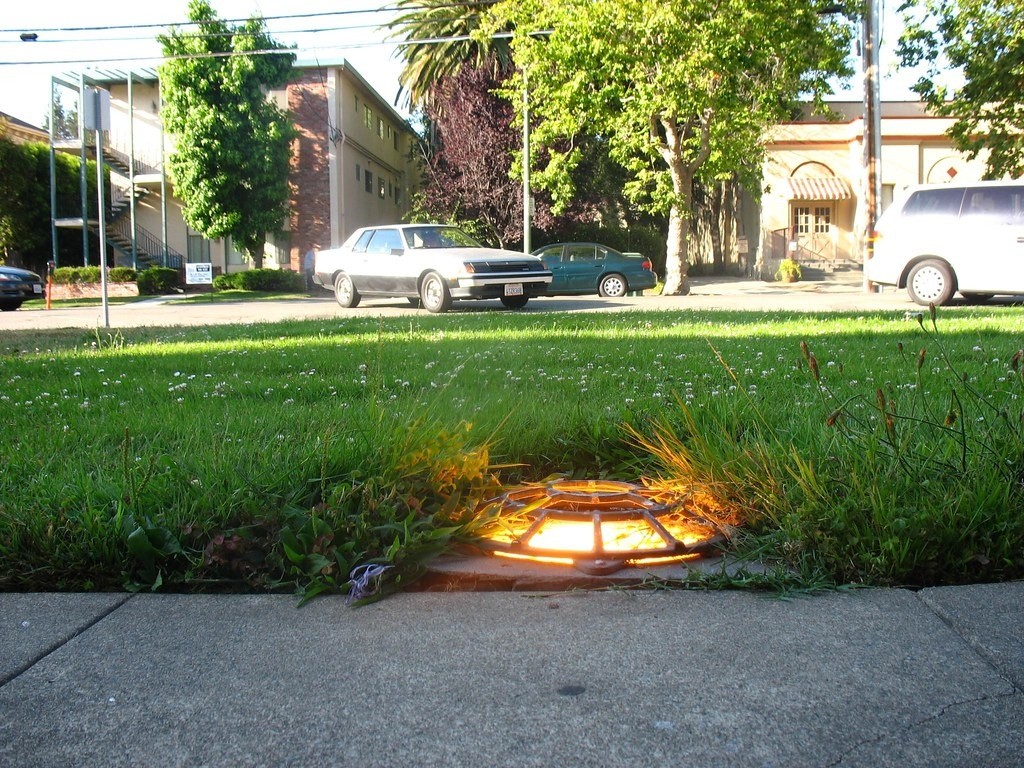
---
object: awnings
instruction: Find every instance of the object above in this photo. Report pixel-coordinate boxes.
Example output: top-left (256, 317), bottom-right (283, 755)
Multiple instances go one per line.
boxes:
top-left (786, 177), bottom-right (851, 200)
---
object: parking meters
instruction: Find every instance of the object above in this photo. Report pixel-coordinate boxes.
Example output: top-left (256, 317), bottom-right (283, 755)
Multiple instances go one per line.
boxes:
top-left (47, 260), bottom-right (56, 309)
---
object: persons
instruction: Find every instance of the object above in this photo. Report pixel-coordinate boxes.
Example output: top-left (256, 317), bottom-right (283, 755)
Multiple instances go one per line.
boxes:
top-left (303, 242), bottom-right (315, 292)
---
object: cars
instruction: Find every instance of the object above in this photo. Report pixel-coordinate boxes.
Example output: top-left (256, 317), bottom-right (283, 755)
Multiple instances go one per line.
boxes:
top-left (0, 265), bottom-right (46, 311)
top-left (868, 180), bottom-right (1024, 307)
top-left (529, 243), bottom-right (657, 298)
top-left (312, 224), bottom-right (553, 313)
